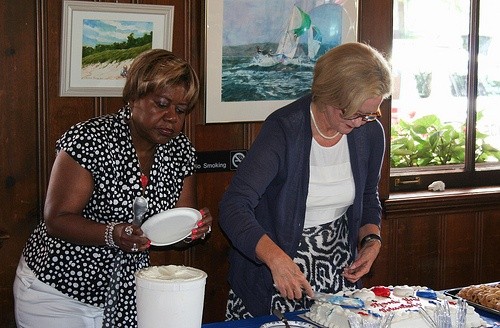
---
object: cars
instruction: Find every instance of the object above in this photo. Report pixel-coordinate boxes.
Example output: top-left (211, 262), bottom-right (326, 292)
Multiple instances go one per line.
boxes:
top-left (388, 36), bottom-right (500, 161)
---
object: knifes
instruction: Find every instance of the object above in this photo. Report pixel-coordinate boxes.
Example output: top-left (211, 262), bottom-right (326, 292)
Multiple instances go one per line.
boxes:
top-left (273, 284), bottom-right (362, 307)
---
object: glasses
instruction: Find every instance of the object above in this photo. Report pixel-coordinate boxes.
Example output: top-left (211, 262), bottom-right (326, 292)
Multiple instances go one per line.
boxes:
top-left (338, 103), bottom-right (381, 122)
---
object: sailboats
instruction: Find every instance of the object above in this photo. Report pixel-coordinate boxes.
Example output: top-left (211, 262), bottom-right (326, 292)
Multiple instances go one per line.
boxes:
top-left (255, 3), bottom-right (327, 72)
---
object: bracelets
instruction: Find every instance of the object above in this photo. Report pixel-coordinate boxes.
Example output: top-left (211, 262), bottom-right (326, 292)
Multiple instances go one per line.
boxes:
top-left (183, 236), bottom-right (192, 244)
top-left (104, 221), bottom-right (122, 249)
top-left (360, 234), bottom-right (382, 248)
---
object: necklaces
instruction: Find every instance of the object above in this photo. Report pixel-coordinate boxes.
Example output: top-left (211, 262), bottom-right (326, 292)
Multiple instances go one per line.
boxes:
top-left (310, 102), bottom-right (340, 139)
top-left (130, 130), bottom-right (158, 189)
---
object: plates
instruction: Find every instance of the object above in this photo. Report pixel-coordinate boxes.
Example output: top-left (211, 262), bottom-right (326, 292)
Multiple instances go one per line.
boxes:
top-left (140, 207), bottom-right (203, 246)
top-left (260, 320), bottom-right (318, 328)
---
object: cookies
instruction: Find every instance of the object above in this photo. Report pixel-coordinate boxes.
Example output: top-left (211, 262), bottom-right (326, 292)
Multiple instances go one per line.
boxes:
top-left (456, 283), bottom-right (500, 313)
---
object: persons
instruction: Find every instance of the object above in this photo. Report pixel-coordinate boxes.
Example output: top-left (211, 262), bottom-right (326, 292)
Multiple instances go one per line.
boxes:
top-left (13, 49), bottom-right (214, 328)
top-left (218, 42), bottom-right (392, 322)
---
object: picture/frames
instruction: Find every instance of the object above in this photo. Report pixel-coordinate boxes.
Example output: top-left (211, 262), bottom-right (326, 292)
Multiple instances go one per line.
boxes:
top-left (196, 0), bottom-right (363, 126)
top-left (58, 0), bottom-right (175, 97)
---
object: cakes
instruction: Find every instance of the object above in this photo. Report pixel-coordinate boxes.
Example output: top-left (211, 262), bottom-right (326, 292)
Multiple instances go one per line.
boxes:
top-left (305, 284), bottom-right (487, 328)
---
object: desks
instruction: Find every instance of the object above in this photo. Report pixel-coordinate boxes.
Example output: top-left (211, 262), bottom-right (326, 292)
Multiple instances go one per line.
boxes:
top-left (201, 283), bottom-right (500, 328)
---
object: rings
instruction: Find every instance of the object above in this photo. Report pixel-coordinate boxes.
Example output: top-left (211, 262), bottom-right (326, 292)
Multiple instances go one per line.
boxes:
top-left (130, 243), bottom-right (138, 253)
top-left (206, 224), bottom-right (212, 234)
top-left (201, 232), bottom-right (206, 240)
top-left (124, 225), bottom-right (134, 236)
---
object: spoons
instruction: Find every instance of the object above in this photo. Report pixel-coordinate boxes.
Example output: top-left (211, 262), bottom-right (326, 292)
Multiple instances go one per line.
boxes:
top-left (132, 197), bottom-right (148, 227)
